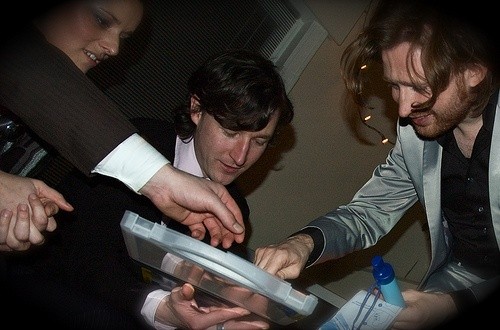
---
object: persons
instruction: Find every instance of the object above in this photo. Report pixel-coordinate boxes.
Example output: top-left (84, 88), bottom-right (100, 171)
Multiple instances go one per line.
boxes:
top-left (0, 0), bottom-right (248, 251)
top-left (18, 49), bottom-right (318, 330)
top-left (0, 0), bottom-right (145, 256)
top-left (252, 1), bottom-right (500, 329)
